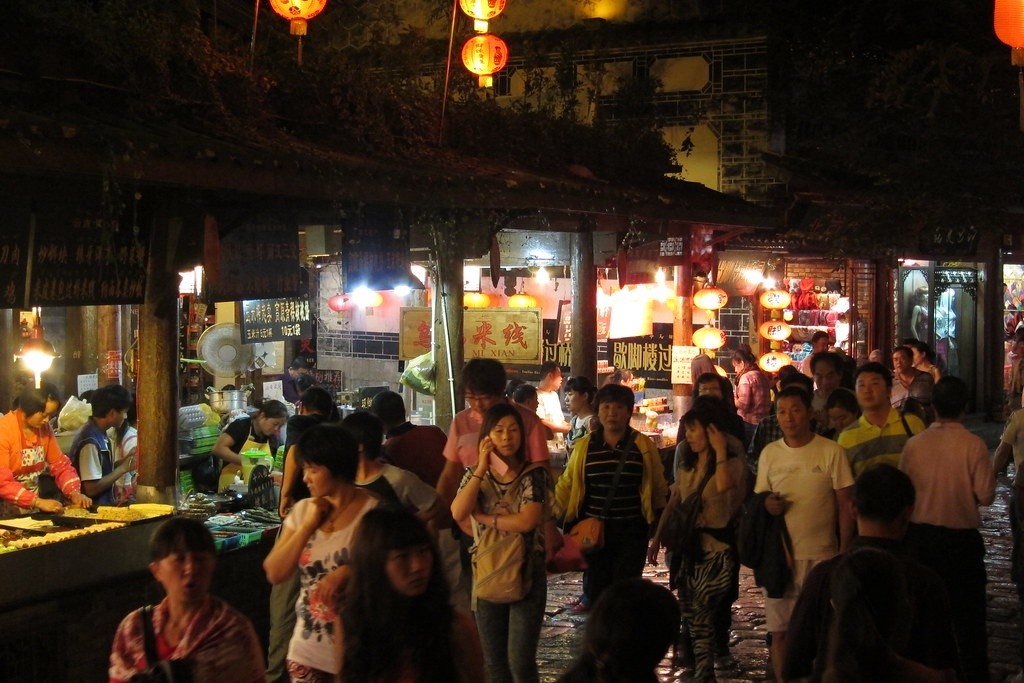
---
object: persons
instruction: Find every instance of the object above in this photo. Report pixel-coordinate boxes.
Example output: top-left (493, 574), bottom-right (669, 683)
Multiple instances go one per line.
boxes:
top-left (911, 286), bottom-right (959, 378)
top-left (264, 354), bottom-right (569, 683)
top-left (1004, 282), bottom-right (1024, 332)
top-left (109, 518), bottom-right (265, 683)
top-left (691, 332), bottom-right (1023, 683)
top-left (835, 313), bottom-right (865, 360)
top-left (556, 372), bottom-right (750, 682)
top-left (0, 382), bottom-right (286, 525)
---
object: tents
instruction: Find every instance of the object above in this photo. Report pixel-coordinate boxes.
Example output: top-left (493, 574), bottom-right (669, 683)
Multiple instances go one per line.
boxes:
top-left (464, 292), bottom-right (491, 309)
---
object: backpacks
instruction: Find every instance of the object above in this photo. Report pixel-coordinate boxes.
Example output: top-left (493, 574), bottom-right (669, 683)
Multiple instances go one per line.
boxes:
top-left (471, 464), bottom-right (550, 604)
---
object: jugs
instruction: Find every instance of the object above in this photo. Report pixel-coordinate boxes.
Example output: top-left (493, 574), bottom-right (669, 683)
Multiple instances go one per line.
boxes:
top-left (240, 447), bottom-right (274, 488)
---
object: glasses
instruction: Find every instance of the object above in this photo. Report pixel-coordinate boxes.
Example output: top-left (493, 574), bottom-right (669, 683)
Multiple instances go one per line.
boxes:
top-left (464, 394), bottom-right (495, 402)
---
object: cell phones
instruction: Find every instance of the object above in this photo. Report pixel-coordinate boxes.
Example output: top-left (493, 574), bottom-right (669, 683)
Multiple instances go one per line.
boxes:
top-left (484, 434), bottom-right (509, 476)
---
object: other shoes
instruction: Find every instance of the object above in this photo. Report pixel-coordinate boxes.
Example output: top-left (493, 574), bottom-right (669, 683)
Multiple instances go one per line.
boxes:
top-left (571, 602), bottom-right (589, 612)
top-left (565, 599), bottom-right (581, 607)
top-left (713, 654), bottom-right (735, 668)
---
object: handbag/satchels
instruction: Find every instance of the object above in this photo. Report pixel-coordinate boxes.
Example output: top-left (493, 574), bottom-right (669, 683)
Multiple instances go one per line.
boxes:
top-left (545, 518), bottom-right (604, 573)
top-left (659, 491), bottom-right (703, 549)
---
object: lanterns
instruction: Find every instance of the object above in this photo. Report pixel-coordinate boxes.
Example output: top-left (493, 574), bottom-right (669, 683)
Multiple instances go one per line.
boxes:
top-left (693, 285), bottom-right (727, 356)
top-left (508, 292), bottom-right (536, 309)
top-left (329, 292), bottom-right (383, 315)
top-left (270, 1), bottom-right (329, 64)
top-left (459, 0), bottom-right (509, 88)
top-left (759, 289), bottom-right (791, 378)
top-left (993, 0), bottom-right (1024, 132)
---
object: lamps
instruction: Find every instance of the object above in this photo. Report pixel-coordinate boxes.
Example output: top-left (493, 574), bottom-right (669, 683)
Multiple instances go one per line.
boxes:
top-left (14, 307), bottom-right (61, 389)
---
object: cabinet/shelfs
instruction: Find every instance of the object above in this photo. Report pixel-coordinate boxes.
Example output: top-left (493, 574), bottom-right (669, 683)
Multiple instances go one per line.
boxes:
top-left (183, 325), bottom-right (214, 405)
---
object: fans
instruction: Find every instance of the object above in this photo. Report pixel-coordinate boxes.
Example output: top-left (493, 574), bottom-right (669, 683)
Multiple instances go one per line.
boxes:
top-left (196, 322), bottom-right (256, 379)
